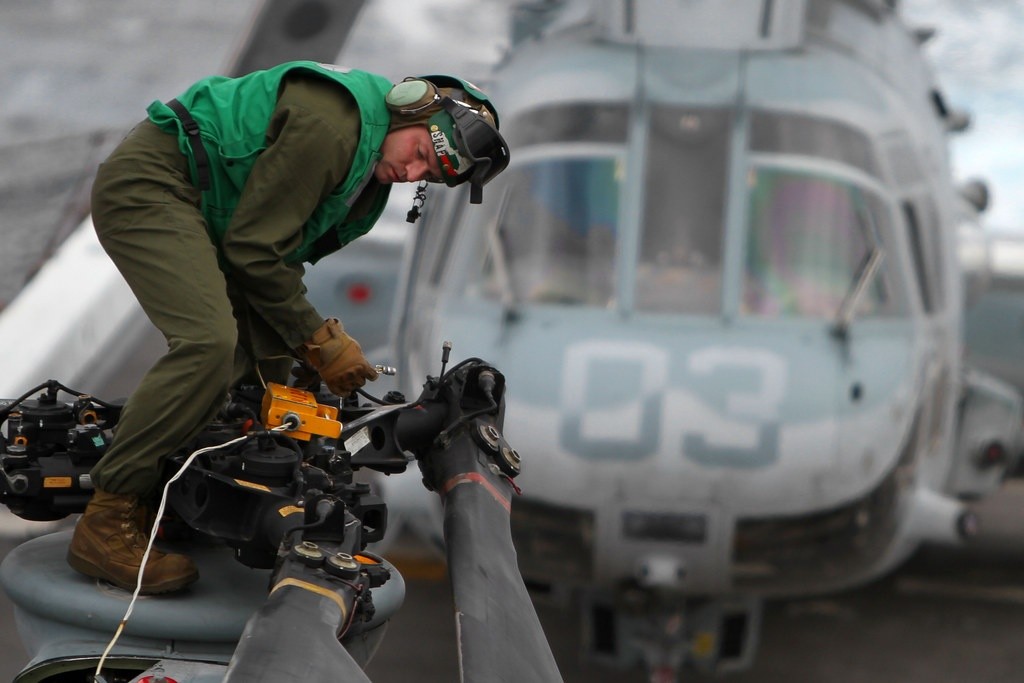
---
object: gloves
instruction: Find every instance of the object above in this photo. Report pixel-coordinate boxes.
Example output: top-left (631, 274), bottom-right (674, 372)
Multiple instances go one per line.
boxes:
top-left (300, 317), bottom-right (379, 397)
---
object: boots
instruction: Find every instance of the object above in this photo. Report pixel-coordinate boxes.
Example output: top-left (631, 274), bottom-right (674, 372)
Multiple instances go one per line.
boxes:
top-left (66, 487), bottom-right (200, 595)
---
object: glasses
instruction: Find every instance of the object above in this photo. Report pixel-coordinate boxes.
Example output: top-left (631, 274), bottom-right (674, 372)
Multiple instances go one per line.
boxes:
top-left (442, 96), bottom-right (511, 186)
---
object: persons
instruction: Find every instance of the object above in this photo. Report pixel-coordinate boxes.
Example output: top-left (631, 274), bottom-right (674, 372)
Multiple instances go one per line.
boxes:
top-left (65, 56), bottom-right (512, 596)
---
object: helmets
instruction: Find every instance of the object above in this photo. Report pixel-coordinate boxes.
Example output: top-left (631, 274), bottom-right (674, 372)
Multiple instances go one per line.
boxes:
top-left (384, 73), bottom-right (500, 188)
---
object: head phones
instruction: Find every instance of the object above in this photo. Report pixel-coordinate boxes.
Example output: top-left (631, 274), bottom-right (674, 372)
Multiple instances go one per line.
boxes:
top-left (384, 76), bottom-right (500, 132)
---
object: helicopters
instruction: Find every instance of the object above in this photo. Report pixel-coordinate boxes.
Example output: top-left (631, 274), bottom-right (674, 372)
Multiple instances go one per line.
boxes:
top-left (295, 3), bottom-right (1024, 682)
